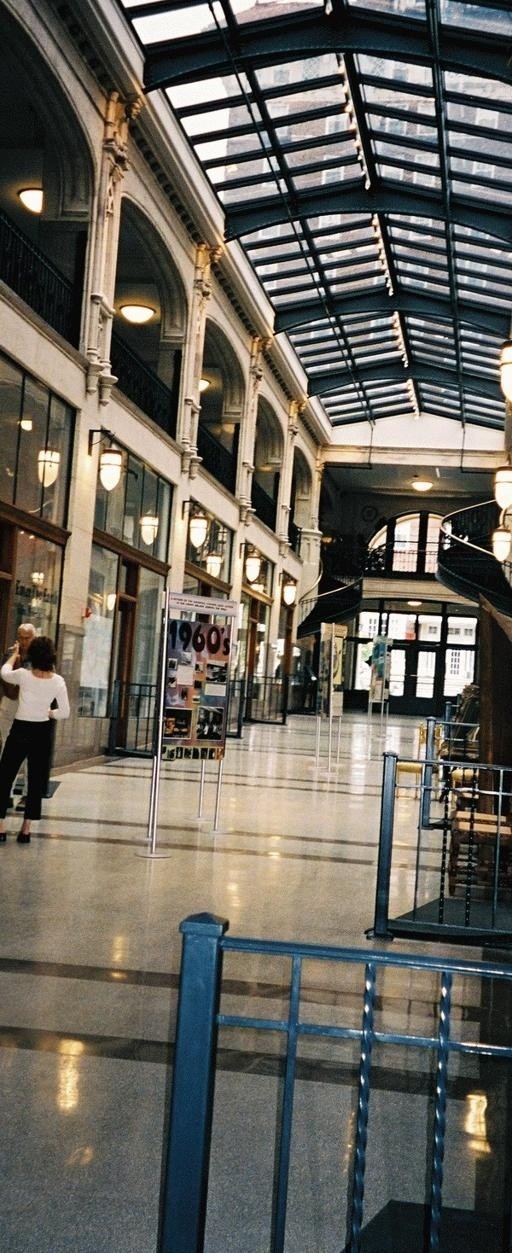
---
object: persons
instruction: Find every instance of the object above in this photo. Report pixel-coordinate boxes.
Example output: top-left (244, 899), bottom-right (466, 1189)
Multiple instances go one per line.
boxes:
top-left (0, 623), bottom-right (35, 810)
top-left (0, 636), bottom-right (71, 844)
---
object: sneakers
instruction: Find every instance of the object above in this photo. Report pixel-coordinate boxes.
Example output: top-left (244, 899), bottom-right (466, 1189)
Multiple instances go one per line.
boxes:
top-left (0, 830), bottom-right (32, 843)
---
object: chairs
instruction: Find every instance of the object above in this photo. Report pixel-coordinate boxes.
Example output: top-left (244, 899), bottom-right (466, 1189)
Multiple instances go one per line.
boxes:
top-left (395, 722), bottom-right (444, 801)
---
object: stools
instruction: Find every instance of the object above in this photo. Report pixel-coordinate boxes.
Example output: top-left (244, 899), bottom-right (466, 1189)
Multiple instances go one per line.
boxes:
top-left (440, 736), bottom-right (479, 802)
top-left (448, 808), bottom-right (511, 897)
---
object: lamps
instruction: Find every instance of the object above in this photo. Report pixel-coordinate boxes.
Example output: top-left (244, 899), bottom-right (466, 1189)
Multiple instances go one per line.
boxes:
top-left (500, 341), bottom-right (512, 405)
top-left (88, 427), bottom-right (125, 492)
top-left (206, 538), bottom-right (228, 576)
top-left (119, 305), bottom-right (156, 327)
top-left (238, 542), bottom-right (261, 584)
top-left (277, 573), bottom-right (296, 606)
top-left (138, 506), bottom-right (158, 546)
top-left (17, 187), bottom-right (43, 212)
top-left (179, 501), bottom-right (209, 548)
top-left (491, 467), bottom-right (511, 562)
top-left (37, 427), bottom-right (65, 487)
